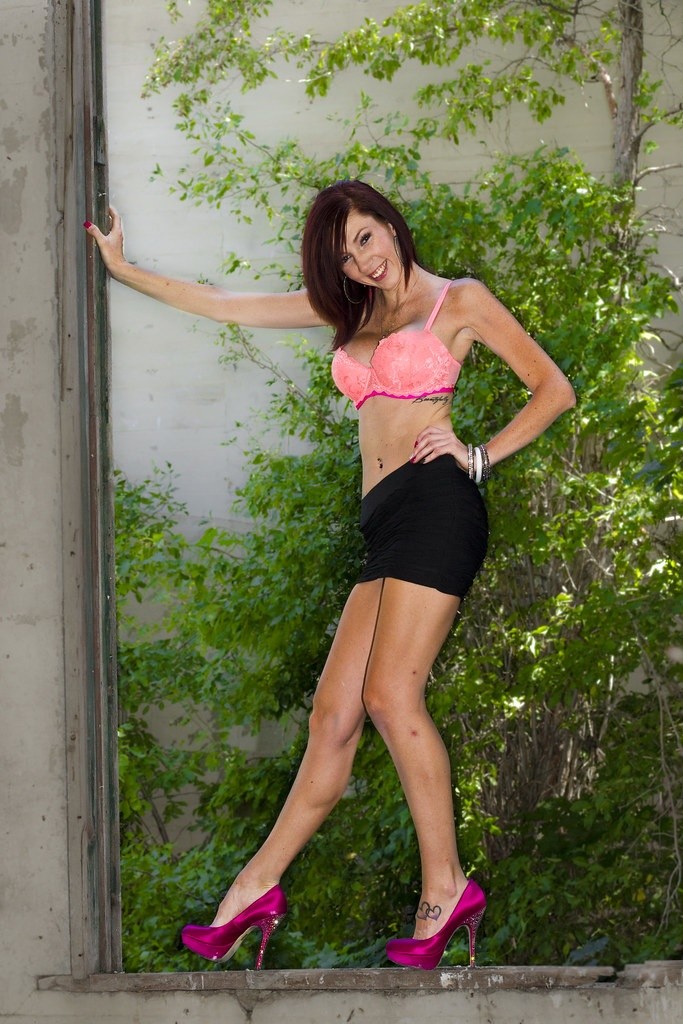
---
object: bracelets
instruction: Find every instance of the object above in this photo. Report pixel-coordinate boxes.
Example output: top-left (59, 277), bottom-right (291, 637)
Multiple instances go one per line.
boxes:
top-left (467, 440), bottom-right (492, 483)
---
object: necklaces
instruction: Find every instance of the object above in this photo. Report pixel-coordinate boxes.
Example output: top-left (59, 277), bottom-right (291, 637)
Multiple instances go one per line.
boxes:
top-left (381, 268), bottom-right (420, 340)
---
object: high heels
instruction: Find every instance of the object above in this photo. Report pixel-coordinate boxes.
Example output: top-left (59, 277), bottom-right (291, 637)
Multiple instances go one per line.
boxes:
top-left (181, 884), bottom-right (288, 971)
top-left (384, 878), bottom-right (487, 970)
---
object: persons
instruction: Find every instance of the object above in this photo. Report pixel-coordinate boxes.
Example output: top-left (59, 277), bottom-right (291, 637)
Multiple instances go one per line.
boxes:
top-left (82, 179), bottom-right (576, 972)
